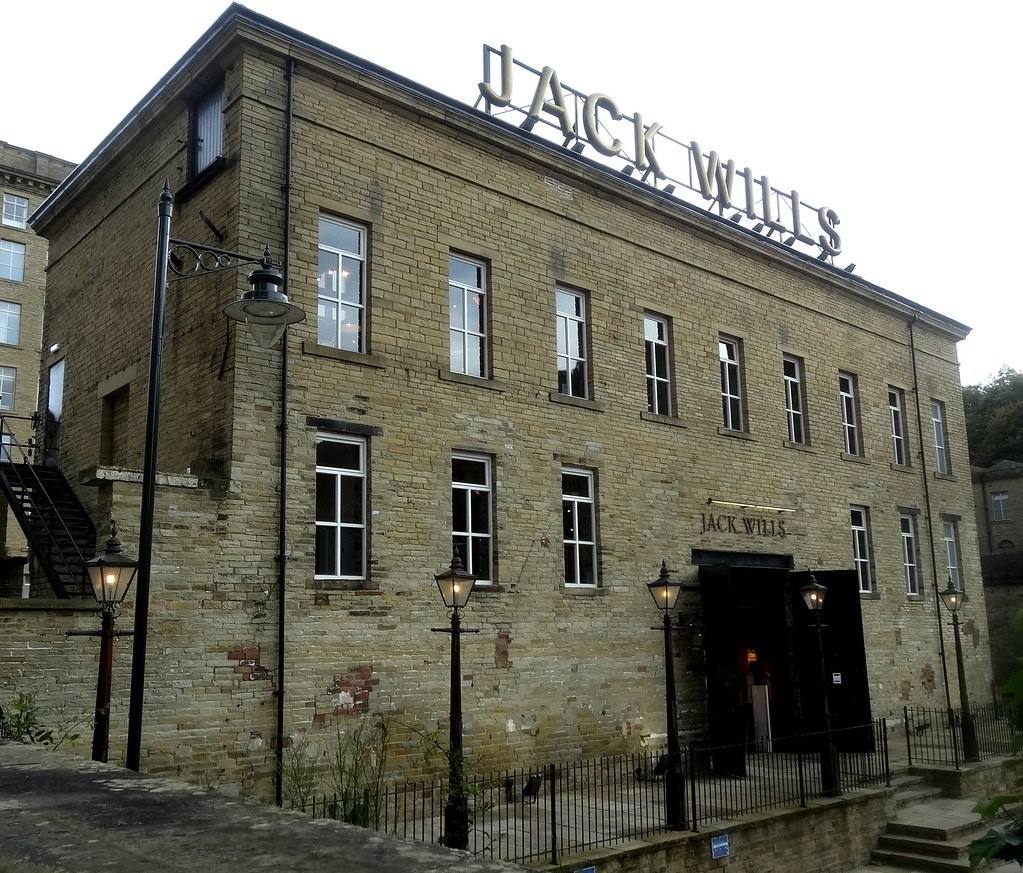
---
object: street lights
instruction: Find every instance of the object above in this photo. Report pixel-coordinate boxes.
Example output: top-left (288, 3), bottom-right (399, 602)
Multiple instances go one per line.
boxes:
top-left (430, 549), bottom-right (484, 850)
top-left (647, 558), bottom-right (692, 831)
top-left (125, 234), bottom-right (312, 779)
top-left (63, 523), bottom-right (139, 766)
top-left (939, 573), bottom-right (984, 763)
top-left (804, 573), bottom-right (847, 797)
